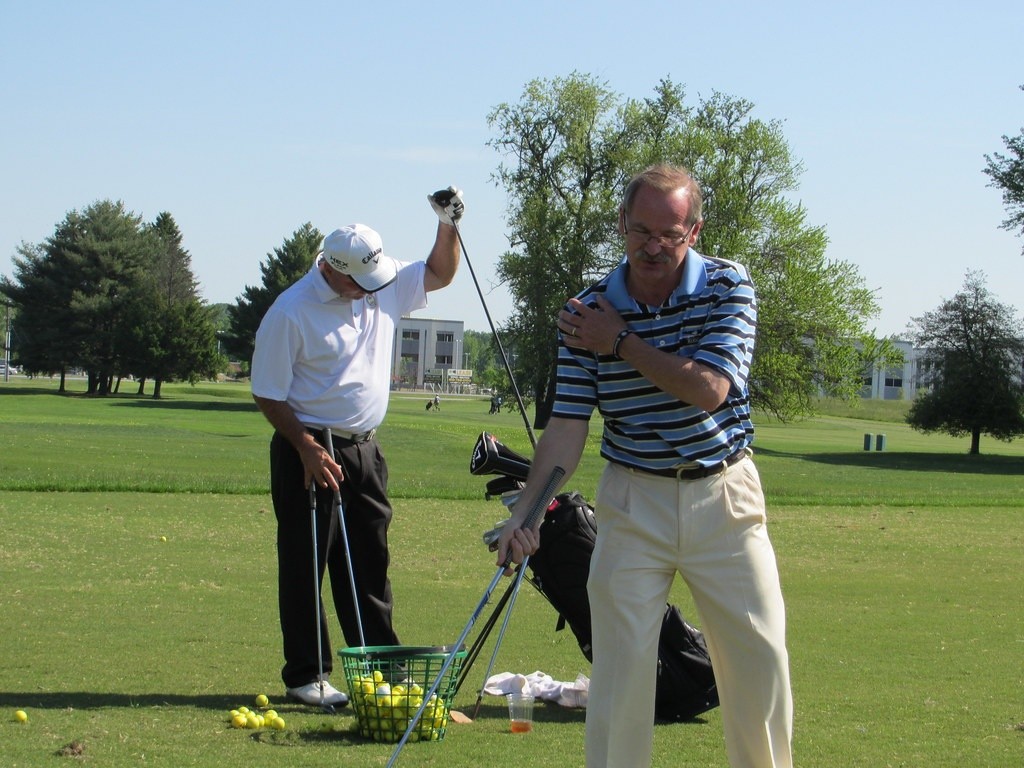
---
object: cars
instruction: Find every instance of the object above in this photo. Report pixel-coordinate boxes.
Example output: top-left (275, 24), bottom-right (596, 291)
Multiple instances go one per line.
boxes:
top-left (0, 365), bottom-right (17, 375)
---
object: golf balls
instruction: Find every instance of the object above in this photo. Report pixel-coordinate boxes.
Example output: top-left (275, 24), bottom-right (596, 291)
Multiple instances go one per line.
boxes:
top-left (229, 695), bottom-right (286, 731)
top-left (348, 670), bottom-right (447, 740)
top-left (303, 428), bottom-right (372, 715)
top-left (14, 710), bottom-right (27, 721)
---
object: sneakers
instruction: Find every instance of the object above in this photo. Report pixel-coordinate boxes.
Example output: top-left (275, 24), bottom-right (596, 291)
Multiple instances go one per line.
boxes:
top-left (287, 681), bottom-right (349, 708)
top-left (396, 678), bottom-right (436, 695)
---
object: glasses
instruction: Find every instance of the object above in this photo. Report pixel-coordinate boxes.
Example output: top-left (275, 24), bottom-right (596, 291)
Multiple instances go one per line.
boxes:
top-left (622, 206), bottom-right (699, 248)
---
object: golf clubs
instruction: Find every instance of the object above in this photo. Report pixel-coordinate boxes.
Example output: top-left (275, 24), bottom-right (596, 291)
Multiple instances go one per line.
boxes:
top-left (432, 190), bottom-right (539, 454)
top-left (383, 468), bottom-right (566, 767)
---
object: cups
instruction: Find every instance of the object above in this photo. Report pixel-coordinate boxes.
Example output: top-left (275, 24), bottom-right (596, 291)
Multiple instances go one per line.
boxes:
top-left (507, 692), bottom-right (534, 732)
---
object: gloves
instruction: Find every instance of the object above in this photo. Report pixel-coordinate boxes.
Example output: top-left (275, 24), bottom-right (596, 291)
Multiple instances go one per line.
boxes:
top-left (427, 186), bottom-right (465, 227)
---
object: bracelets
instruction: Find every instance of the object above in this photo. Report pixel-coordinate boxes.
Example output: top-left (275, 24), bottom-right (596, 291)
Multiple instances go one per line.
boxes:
top-left (612, 329), bottom-right (636, 360)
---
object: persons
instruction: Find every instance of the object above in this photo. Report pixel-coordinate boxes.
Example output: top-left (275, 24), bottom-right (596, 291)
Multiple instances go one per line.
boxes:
top-left (249, 184), bottom-right (466, 711)
top-left (499, 163), bottom-right (796, 768)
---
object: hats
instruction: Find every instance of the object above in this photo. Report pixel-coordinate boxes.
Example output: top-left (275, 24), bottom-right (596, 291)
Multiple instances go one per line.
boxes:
top-left (323, 224), bottom-right (399, 294)
